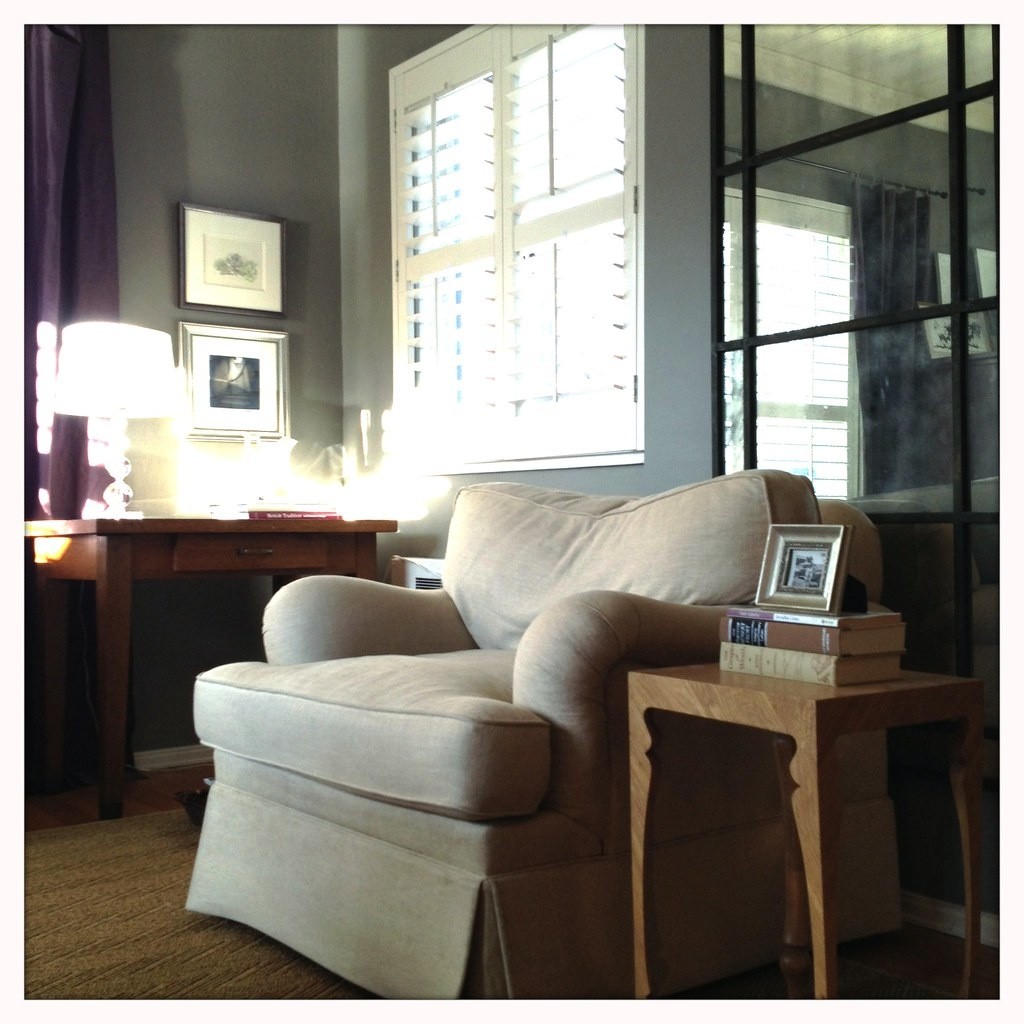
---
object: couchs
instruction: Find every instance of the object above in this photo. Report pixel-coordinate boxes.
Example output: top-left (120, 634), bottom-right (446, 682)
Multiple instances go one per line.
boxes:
top-left (185, 482), bottom-right (904, 1000)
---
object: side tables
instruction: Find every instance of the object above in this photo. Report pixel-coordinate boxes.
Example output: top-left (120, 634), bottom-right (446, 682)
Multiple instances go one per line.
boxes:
top-left (626, 656), bottom-right (984, 1000)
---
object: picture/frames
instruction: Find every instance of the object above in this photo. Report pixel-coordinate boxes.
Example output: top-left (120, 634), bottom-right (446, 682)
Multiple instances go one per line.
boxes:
top-left (178, 321), bottom-right (290, 443)
top-left (755, 526), bottom-right (854, 617)
top-left (178, 201), bottom-right (287, 318)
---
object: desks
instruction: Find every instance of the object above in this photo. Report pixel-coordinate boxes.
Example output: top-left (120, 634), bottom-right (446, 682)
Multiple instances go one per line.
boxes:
top-left (24, 518), bottom-right (398, 821)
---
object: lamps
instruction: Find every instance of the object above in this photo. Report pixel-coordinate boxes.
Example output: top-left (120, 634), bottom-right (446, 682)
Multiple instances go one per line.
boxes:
top-left (49, 320), bottom-right (183, 519)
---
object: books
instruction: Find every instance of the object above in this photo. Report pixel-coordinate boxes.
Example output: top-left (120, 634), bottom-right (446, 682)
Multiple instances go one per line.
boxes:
top-left (727, 602), bottom-right (905, 630)
top-left (718, 613), bottom-right (906, 689)
top-left (208, 504), bottom-right (343, 523)
